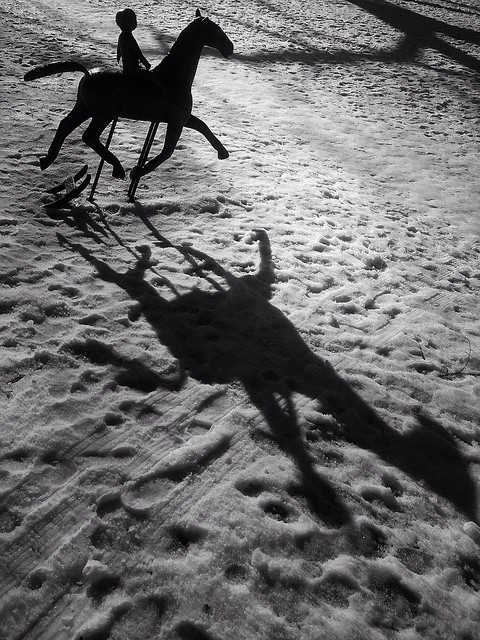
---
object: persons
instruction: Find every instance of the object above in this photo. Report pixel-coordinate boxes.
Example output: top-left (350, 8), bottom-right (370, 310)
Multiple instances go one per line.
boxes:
top-left (115, 8), bottom-right (156, 80)
